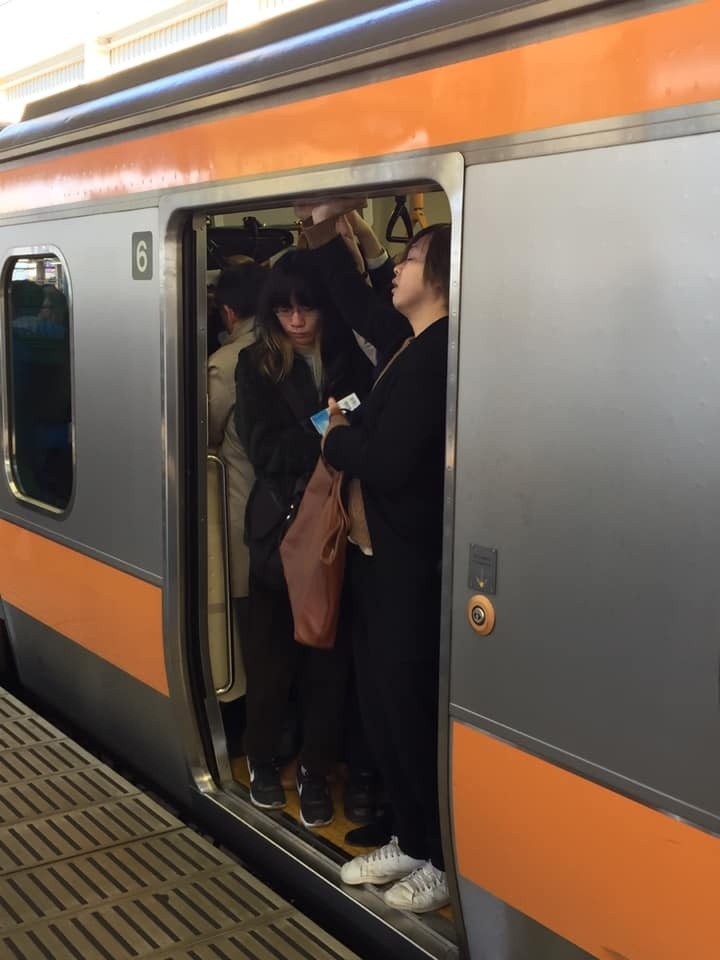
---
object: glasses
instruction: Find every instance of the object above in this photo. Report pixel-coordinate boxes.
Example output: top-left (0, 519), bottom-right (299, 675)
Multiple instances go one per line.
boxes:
top-left (274, 304), bottom-right (319, 316)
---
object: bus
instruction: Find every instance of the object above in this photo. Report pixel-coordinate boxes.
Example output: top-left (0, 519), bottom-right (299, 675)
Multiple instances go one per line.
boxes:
top-left (0, 0), bottom-right (719, 960)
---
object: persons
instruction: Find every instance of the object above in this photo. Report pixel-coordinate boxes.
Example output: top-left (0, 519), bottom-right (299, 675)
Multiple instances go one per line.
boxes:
top-left (10, 279), bottom-right (68, 343)
top-left (208, 206), bottom-right (393, 849)
top-left (322, 210), bottom-right (452, 912)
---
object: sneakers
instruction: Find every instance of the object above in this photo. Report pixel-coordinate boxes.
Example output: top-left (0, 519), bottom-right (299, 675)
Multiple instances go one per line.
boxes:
top-left (296, 759), bottom-right (336, 827)
top-left (246, 752), bottom-right (288, 809)
top-left (340, 835), bottom-right (427, 885)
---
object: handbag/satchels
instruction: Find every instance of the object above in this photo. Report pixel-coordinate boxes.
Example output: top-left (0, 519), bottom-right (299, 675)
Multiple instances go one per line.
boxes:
top-left (278, 454), bottom-right (351, 649)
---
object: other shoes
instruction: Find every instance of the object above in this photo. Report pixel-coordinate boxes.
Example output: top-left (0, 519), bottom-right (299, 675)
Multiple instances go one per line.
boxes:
top-left (338, 761), bottom-right (373, 821)
top-left (344, 818), bottom-right (394, 848)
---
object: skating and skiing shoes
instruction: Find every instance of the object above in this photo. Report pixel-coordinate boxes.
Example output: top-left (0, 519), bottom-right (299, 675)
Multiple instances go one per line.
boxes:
top-left (383, 858), bottom-right (450, 913)
